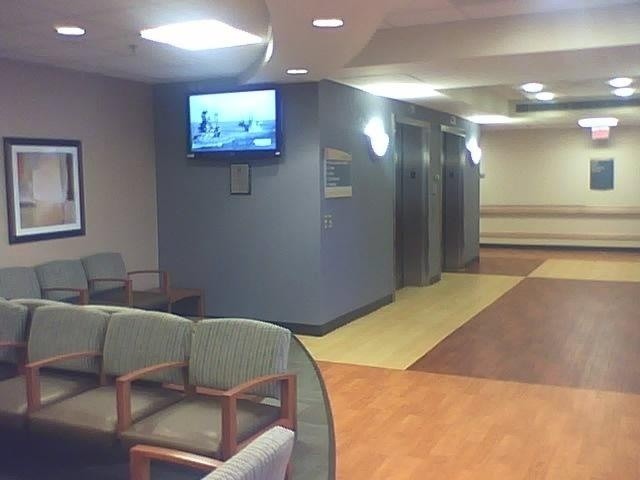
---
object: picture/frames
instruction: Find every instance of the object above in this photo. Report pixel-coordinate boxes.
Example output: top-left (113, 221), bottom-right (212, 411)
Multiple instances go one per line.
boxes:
top-left (4, 137), bottom-right (86, 245)
top-left (230, 163), bottom-right (251, 195)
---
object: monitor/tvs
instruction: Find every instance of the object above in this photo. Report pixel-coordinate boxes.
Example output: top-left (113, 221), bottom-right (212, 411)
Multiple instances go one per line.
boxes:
top-left (185, 84), bottom-right (283, 160)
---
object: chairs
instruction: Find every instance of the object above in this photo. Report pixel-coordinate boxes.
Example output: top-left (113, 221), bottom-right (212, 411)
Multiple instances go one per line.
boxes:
top-left (0, 305), bottom-right (111, 480)
top-left (116, 318), bottom-right (292, 480)
top-left (23, 310), bottom-right (194, 480)
top-left (0, 253), bottom-right (205, 317)
top-left (0, 296), bottom-right (26, 381)
top-left (128, 425), bottom-right (296, 480)
